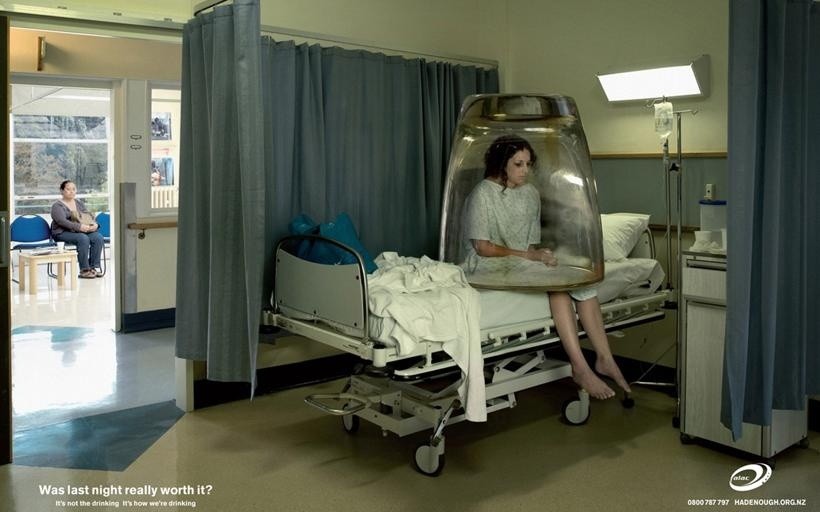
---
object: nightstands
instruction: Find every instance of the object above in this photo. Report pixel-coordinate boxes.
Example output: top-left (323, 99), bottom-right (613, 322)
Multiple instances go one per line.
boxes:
top-left (678, 249), bottom-right (811, 470)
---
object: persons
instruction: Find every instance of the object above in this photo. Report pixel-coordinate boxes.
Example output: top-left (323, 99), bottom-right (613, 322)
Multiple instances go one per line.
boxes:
top-left (459, 134), bottom-right (634, 401)
top-left (49, 179), bottom-right (105, 279)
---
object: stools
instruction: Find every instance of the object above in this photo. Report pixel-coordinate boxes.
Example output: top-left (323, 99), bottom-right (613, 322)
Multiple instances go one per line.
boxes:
top-left (16, 251), bottom-right (79, 295)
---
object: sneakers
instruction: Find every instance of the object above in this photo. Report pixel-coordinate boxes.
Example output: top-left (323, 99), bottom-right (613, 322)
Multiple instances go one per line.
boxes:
top-left (76, 267), bottom-right (103, 278)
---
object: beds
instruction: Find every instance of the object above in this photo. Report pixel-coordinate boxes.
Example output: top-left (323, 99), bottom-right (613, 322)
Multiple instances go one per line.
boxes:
top-left (260, 226), bottom-right (674, 477)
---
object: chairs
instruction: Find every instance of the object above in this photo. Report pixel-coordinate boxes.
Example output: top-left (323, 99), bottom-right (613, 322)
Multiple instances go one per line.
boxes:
top-left (10, 210), bottom-right (110, 284)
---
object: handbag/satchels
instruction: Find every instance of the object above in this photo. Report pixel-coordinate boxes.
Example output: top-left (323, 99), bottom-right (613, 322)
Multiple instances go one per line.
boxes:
top-left (70, 210), bottom-right (98, 233)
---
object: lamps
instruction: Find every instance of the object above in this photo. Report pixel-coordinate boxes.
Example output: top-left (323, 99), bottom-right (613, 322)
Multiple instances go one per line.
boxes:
top-left (596, 54), bottom-right (710, 104)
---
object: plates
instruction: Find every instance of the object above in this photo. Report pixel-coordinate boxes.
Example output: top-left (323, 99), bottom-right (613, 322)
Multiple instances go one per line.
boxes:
top-left (31, 250), bottom-right (50, 255)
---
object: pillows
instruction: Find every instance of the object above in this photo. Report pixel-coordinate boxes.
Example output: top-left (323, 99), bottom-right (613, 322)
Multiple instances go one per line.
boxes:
top-left (597, 212), bottom-right (654, 264)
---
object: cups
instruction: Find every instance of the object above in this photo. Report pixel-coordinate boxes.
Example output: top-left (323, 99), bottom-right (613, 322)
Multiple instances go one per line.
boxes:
top-left (693, 231), bottom-right (711, 244)
top-left (57, 241), bottom-right (64, 253)
top-left (438, 92), bottom-right (606, 293)
